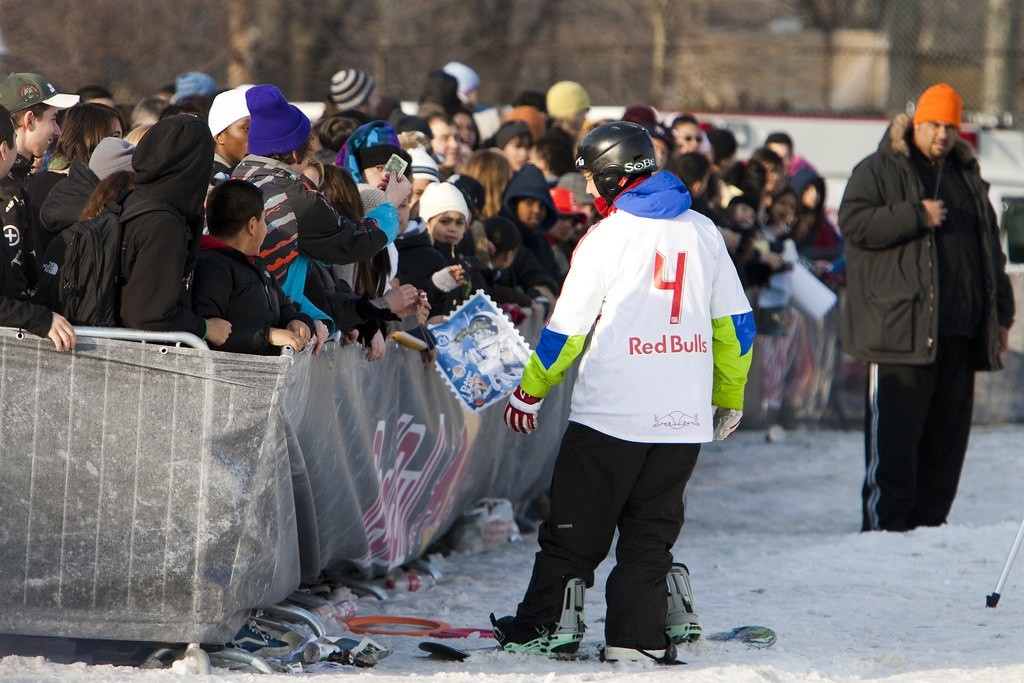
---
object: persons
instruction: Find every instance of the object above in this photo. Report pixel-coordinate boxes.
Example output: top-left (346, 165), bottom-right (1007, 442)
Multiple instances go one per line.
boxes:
top-left (493, 121), bottom-right (757, 663)
top-left (0, 63), bottom-right (853, 360)
top-left (837, 82), bottom-right (1015, 532)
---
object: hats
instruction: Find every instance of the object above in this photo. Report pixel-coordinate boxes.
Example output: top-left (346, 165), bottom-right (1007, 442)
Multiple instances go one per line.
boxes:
top-left (354, 125), bottom-right (401, 172)
top-left (545, 80), bottom-right (590, 118)
top-left (913, 83), bottom-right (962, 132)
top-left (624, 106), bottom-right (655, 130)
top-left (407, 148), bottom-right (439, 182)
top-left (176, 71), bottom-right (214, 97)
top-left (0, 72), bottom-right (80, 113)
top-left (88, 137), bottom-right (136, 181)
top-left (245, 85), bottom-right (310, 156)
top-left (329, 68), bottom-right (373, 110)
top-left (444, 62), bottom-right (479, 94)
top-left (420, 182), bottom-right (468, 222)
top-left (207, 89), bottom-right (250, 136)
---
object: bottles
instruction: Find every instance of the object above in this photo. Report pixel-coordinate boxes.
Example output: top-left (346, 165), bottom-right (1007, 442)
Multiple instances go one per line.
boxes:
top-left (303, 642), bottom-right (343, 664)
top-left (386, 574), bottom-right (434, 592)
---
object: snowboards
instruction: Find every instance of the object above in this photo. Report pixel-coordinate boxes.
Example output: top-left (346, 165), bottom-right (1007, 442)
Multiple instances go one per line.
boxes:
top-left (418, 626), bottom-right (777, 662)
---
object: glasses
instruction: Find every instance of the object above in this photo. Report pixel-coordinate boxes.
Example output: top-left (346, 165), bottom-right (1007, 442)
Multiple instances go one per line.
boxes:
top-left (683, 135), bottom-right (704, 144)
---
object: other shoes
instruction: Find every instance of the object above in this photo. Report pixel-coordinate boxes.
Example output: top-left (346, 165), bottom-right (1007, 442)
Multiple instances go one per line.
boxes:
top-left (495, 614), bottom-right (578, 660)
top-left (602, 633), bottom-right (670, 660)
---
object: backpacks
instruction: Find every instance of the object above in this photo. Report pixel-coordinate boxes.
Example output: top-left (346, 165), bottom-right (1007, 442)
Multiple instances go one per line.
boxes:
top-left (35, 188), bottom-right (187, 326)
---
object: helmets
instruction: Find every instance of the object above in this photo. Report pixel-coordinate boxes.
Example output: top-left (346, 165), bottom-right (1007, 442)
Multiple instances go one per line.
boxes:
top-left (574, 122), bottom-right (658, 198)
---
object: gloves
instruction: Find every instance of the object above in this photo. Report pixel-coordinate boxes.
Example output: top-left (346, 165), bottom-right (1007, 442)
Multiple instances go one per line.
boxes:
top-left (712, 406), bottom-right (742, 440)
top-left (503, 386), bottom-right (545, 434)
